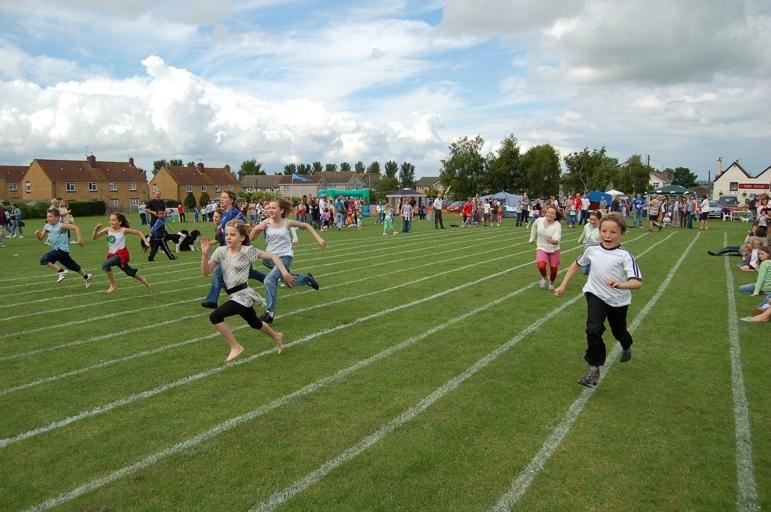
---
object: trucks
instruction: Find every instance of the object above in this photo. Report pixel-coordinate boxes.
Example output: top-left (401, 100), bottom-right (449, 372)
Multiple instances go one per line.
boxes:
top-left (619, 195), bottom-right (634, 211)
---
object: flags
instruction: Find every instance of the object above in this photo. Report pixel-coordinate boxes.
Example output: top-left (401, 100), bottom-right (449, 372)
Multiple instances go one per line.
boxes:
top-left (291, 173), bottom-right (311, 183)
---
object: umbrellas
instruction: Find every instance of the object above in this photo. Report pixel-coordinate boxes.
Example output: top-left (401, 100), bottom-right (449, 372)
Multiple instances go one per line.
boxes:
top-left (385, 187), bottom-right (427, 198)
top-left (646, 184), bottom-right (698, 195)
top-left (605, 189), bottom-right (624, 196)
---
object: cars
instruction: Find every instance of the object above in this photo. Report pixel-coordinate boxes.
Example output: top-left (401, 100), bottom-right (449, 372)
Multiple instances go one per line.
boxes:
top-left (693, 197), bottom-right (742, 219)
top-left (0, 199), bottom-right (38, 206)
top-left (426, 198), bottom-right (448, 206)
top-left (448, 201), bottom-right (467, 212)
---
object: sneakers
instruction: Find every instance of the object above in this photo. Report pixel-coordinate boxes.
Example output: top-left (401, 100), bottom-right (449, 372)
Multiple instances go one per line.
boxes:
top-left (740, 307), bottom-right (770, 323)
top-left (83, 273), bottom-right (94, 289)
top-left (55, 269), bottom-right (69, 283)
top-left (201, 300), bottom-right (219, 310)
top-left (382, 232), bottom-right (390, 236)
top-left (257, 311), bottom-right (275, 324)
top-left (69, 240), bottom-right (79, 245)
top-left (460, 221), bottom-right (503, 228)
top-left (540, 276), bottom-right (548, 289)
top-left (305, 271), bottom-right (322, 291)
top-left (618, 345), bottom-right (634, 363)
top-left (4, 232), bottom-right (25, 240)
top-left (706, 249), bottom-right (718, 257)
top-left (549, 279), bottom-right (556, 291)
top-left (393, 231), bottom-right (400, 235)
top-left (575, 367), bottom-right (602, 390)
top-left (631, 225), bottom-right (711, 234)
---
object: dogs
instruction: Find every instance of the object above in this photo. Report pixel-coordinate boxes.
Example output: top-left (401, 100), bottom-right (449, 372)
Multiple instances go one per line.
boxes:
top-left (140, 229), bottom-right (202, 255)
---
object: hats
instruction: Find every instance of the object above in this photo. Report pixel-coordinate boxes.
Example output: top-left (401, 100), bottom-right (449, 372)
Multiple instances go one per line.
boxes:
top-left (651, 193), bottom-right (658, 199)
